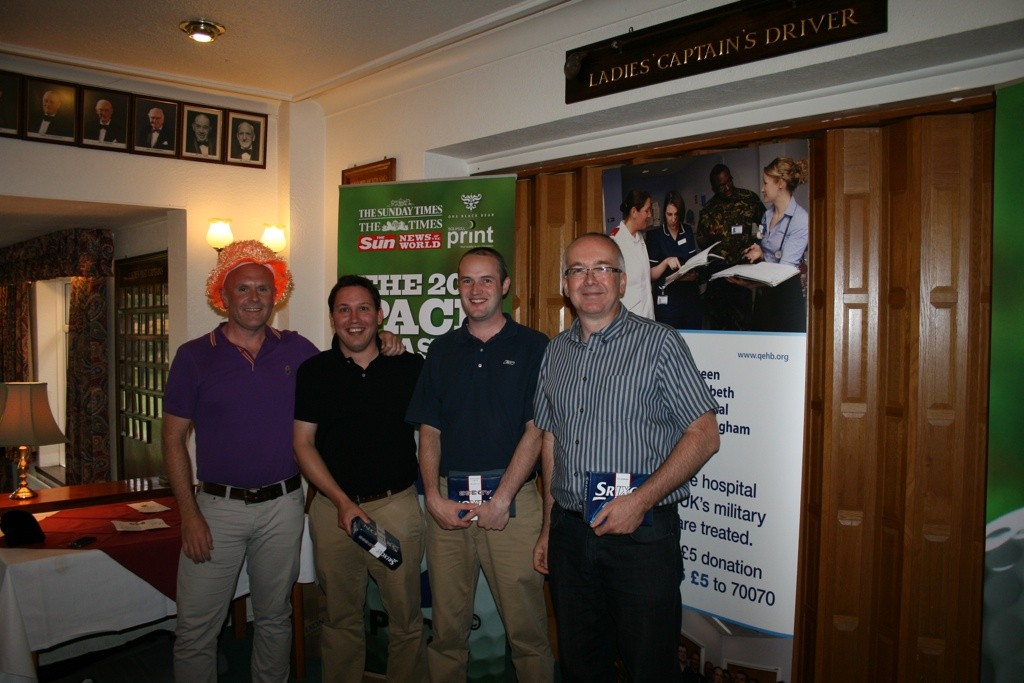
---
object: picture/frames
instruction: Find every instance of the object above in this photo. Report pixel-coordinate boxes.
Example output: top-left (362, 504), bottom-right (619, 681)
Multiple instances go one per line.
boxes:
top-left (23, 75), bottom-right (77, 145)
top-left (80, 84), bottom-right (132, 152)
top-left (180, 102), bottom-right (226, 164)
top-left (0, 70), bottom-right (24, 137)
top-left (223, 109), bottom-right (269, 169)
top-left (132, 95), bottom-right (179, 159)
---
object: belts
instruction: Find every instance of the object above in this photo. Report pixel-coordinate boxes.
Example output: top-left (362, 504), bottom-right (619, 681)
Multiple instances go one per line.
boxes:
top-left (318, 479), bottom-right (415, 505)
top-left (203, 472), bottom-right (302, 505)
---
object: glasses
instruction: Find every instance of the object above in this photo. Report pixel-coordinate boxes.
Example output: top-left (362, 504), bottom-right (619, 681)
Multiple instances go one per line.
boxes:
top-left (564, 267), bottom-right (622, 277)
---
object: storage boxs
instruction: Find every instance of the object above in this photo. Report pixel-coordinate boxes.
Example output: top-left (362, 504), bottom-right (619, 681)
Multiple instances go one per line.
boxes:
top-left (353, 515), bottom-right (403, 570)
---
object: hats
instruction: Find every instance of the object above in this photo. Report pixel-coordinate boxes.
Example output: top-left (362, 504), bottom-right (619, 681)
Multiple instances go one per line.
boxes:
top-left (204, 240), bottom-right (295, 313)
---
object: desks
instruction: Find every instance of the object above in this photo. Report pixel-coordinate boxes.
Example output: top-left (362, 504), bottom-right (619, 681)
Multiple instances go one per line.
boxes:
top-left (1, 496), bottom-right (317, 682)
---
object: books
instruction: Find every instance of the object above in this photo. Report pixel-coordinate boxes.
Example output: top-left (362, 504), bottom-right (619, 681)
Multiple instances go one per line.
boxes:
top-left (583, 470), bottom-right (651, 525)
top-left (447, 474), bottom-right (516, 521)
top-left (350, 516), bottom-right (404, 571)
top-left (664, 240), bottom-right (724, 289)
top-left (708, 261), bottom-right (801, 288)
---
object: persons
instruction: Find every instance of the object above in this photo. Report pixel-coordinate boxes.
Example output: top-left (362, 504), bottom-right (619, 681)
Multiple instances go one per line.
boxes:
top-left (293, 274), bottom-right (427, 683)
top-left (609, 189), bottom-right (655, 321)
top-left (532, 232), bottom-right (721, 683)
top-left (25, 79), bottom-right (260, 165)
top-left (415, 248), bottom-right (552, 682)
top-left (746, 156), bottom-right (808, 333)
top-left (644, 191), bottom-right (697, 329)
top-left (161, 242), bottom-right (405, 683)
top-left (697, 164), bottom-right (767, 331)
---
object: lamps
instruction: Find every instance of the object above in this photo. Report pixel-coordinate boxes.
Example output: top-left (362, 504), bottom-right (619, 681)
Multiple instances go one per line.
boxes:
top-left (179, 18), bottom-right (226, 43)
top-left (207, 219), bottom-right (289, 257)
top-left (0, 381), bottom-right (68, 501)
